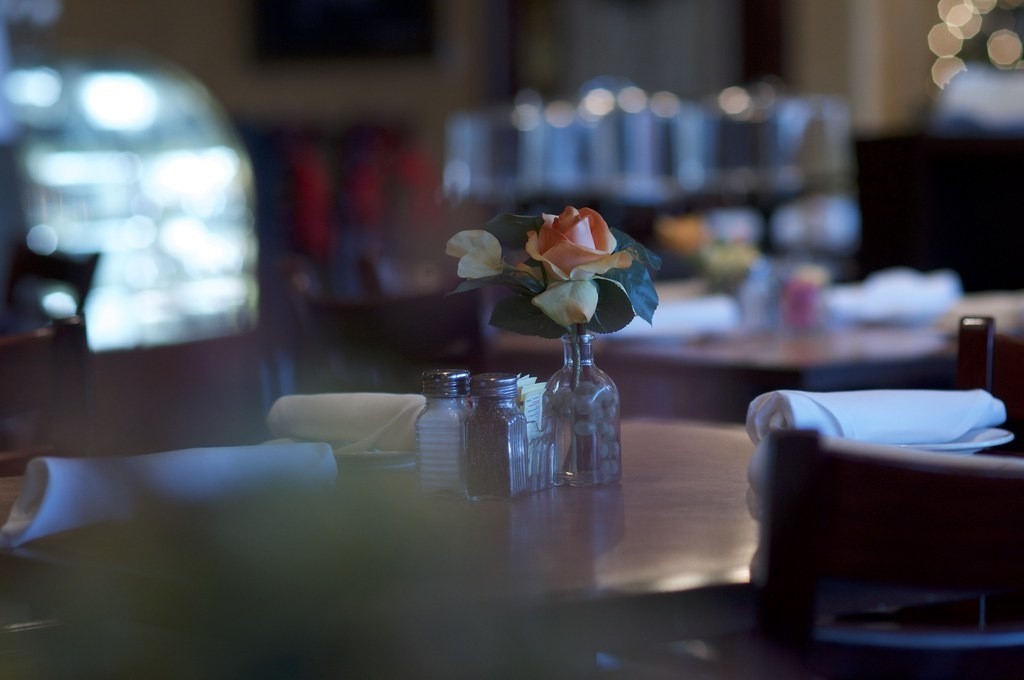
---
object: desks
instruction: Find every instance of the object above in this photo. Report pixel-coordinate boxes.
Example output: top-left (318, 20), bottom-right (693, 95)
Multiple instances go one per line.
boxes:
top-left (494, 290), bottom-right (1023, 428)
top-left (2, 413), bottom-right (813, 680)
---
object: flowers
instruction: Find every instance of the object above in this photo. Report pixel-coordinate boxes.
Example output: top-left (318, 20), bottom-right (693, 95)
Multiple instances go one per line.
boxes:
top-left (698, 242), bottom-right (760, 291)
top-left (446, 205), bottom-right (665, 476)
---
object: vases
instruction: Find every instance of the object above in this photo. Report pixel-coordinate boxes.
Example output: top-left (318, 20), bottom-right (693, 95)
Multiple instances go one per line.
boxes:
top-left (542, 334), bottom-right (623, 488)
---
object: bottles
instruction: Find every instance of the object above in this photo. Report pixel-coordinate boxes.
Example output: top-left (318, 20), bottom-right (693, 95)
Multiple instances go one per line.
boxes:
top-left (415, 368), bottom-right (471, 492)
top-left (468, 373), bottom-right (525, 497)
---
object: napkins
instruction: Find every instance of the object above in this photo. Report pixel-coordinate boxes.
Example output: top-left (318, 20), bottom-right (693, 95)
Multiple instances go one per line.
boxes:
top-left (1, 440), bottom-right (342, 546)
top-left (743, 387), bottom-right (1007, 490)
top-left (598, 293), bottom-right (739, 340)
top-left (267, 393), bottom-right (425, 456)
top-left (819, 269), bottom-right (962, 329)
